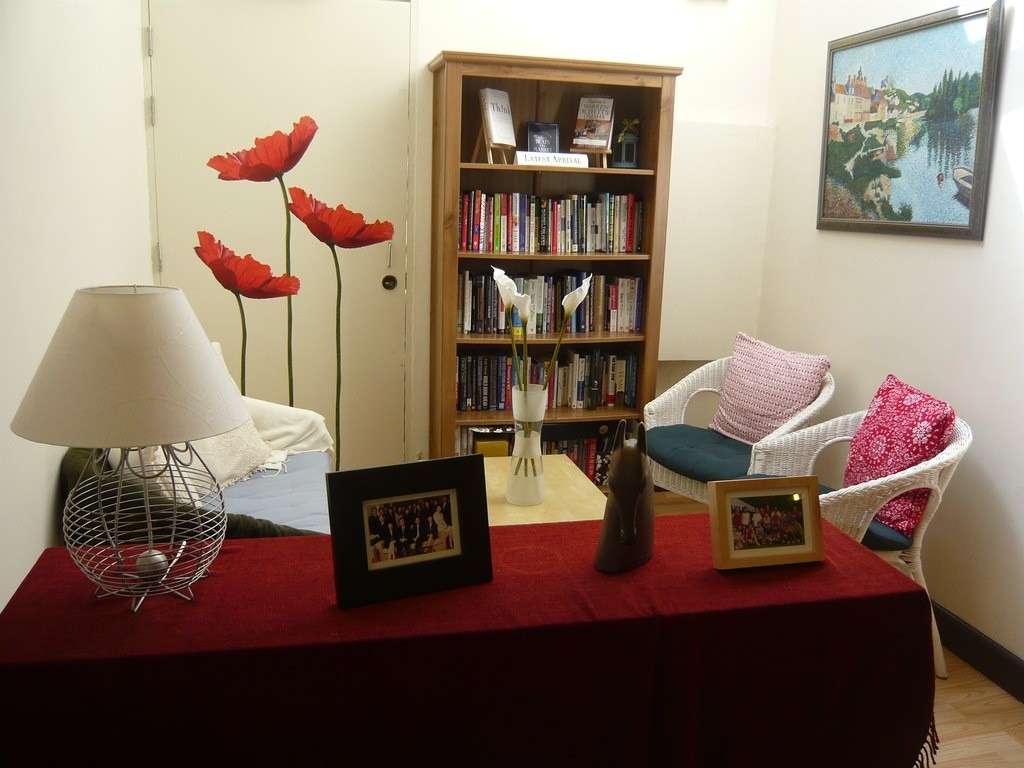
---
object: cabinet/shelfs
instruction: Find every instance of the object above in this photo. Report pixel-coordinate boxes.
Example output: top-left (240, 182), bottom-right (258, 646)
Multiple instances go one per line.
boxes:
top-left (428, 50), bottom-right (684, 507)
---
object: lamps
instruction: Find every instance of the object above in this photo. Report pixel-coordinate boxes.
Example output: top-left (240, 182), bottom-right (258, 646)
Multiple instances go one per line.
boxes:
top-left (9, 285), bottom-right (250, 613)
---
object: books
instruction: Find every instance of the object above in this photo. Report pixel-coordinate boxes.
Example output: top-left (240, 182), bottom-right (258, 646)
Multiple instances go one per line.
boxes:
top-left (456, 88), bottom-right (645, 488)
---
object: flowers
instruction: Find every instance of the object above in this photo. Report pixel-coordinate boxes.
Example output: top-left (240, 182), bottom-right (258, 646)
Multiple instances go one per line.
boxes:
top-left (193, 229), bottom-right (300, 395)
top-left (490, 265), bottom-right (592, 392)
top-left (204, 115), bottom-right (319, 407)
top-left (287, 186), bottom-right (394, 470)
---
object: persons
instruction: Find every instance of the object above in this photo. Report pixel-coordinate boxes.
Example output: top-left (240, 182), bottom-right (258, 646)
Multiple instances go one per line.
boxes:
top-left (732, 502), bottom-right (804, 550)
top-left (369, 495), bottom-right (454, 564)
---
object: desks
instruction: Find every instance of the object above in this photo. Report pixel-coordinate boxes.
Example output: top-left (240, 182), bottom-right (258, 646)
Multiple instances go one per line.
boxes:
top-left (0, 513), bottom-right (940, 768)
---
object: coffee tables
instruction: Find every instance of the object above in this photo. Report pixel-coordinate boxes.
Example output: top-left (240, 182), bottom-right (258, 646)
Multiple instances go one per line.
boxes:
top-left (416, 454), bottom-right (607, 526)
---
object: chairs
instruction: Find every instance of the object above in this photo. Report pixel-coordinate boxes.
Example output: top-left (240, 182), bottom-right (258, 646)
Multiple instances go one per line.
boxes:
top-left (753, 410), bottom-right (972, 680)
top-left (643, 355), bottom-right (836, 506)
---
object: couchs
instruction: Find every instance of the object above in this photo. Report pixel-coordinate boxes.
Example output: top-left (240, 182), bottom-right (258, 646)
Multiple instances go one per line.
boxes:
top-left (60, 397), bottom-right (335, 546)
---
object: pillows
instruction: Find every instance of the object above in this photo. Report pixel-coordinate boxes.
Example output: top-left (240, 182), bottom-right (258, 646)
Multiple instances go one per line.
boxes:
top-left (707, 331), bottom-right (831, 446)
top-left (102, 446), bottom-right (203, 508)
top-left (172, 341), bottom-right (272, 496)
top-left (842, 375), bottom-right (955, 538)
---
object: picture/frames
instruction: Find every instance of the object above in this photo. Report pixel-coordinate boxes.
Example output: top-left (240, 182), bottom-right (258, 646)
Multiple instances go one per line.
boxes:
top-left (817, 0), bottom-right (1006, 241)
top-left (707, 476), bottom-right (825, 570)
top-left (325, 453), bottom-right (494, 610)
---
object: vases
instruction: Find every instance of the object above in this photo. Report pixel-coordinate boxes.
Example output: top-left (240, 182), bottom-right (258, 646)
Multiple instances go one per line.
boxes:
top-left (506, 383), bottom-right (548, 507)
